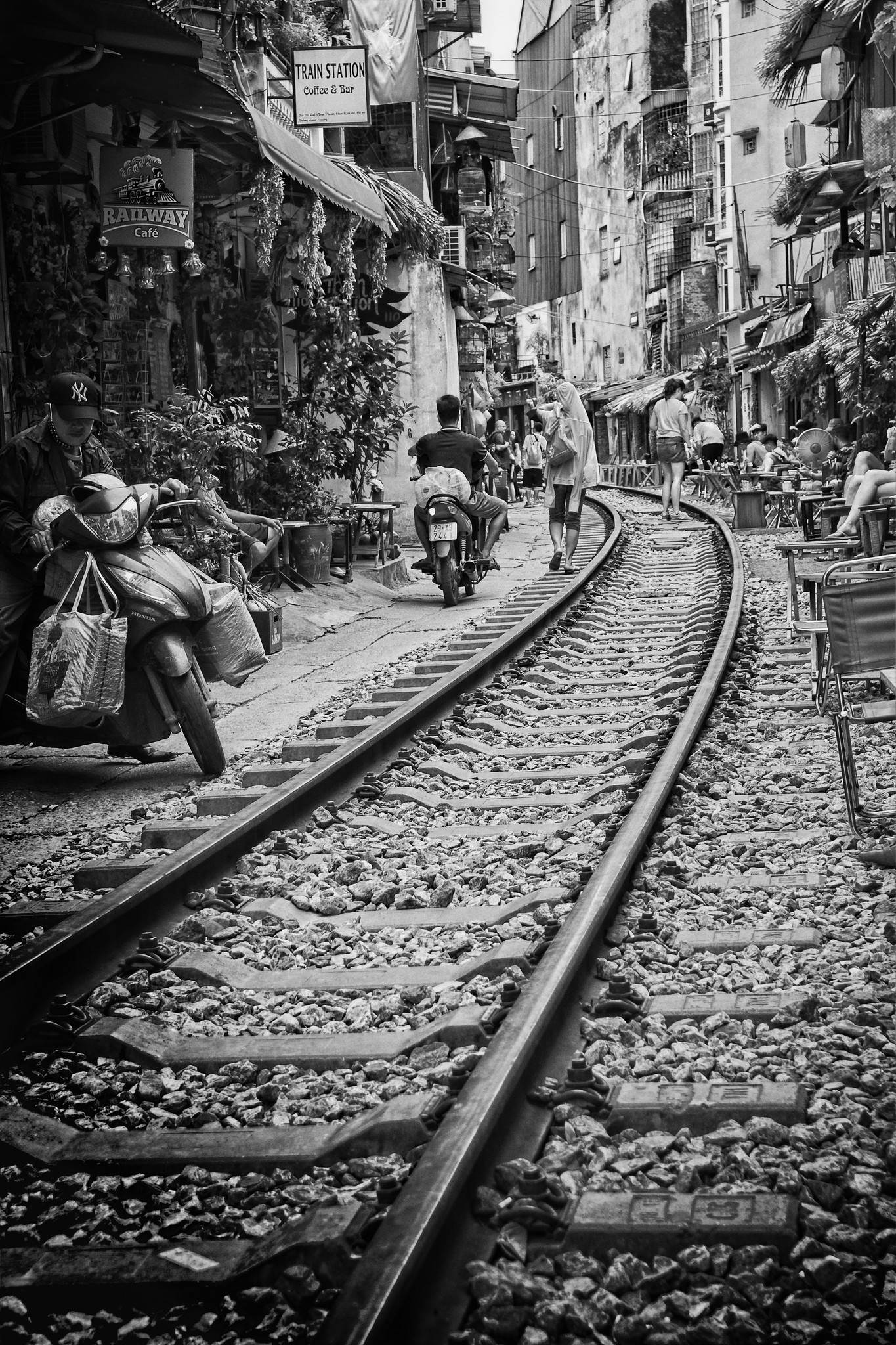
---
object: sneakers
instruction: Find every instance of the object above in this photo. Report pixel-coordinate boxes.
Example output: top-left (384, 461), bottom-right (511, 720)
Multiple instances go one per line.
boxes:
top-left (670, 511), bottom-right (693, 522)
top-left (524, 501), bottom-right (532, 508)
top-left (532, 501), bottom-right (542, 507)
top-left (662, 512), bottom-right (671, 521)
top-left (507, 495), bottom-right (524, 503)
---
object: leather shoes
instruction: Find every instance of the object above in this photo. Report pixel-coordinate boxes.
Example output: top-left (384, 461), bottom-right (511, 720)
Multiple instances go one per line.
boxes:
top-left (107, 743), bottom-right (176, 763)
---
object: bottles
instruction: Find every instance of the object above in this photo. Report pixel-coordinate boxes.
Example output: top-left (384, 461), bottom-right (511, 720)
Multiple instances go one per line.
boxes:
top-left (718, 457), bottom-right (721, 472)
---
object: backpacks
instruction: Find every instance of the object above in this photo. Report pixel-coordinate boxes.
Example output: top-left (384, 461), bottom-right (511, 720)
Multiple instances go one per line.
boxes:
top-left (486, 431), bottom-right (506, 469)
top-left (526, 434), bottom-right (544, 465)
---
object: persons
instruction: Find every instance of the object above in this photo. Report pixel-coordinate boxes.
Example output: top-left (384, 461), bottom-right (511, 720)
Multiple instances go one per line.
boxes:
top-left (480, 363), bottom-right (896, 574)
top-left (172, 442), bottom-right (285, 579)
top-left (0, 370), bottom-right (192, 764)
top-left (266, 437), bottom-right (302, 495)
top-left (408, 393), bottom-right (509, 572)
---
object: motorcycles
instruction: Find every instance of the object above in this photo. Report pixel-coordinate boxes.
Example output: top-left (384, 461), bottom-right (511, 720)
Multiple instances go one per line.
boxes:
top-left (0, 478), bottom-right (233, 777)
top-left (409, 467), bottom-right (497, 607)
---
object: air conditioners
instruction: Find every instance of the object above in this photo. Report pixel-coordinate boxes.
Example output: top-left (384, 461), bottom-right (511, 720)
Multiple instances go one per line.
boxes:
top-left (703, 222), bottom-right (719, 247)
top-left (439, 225), bottom-right (467, 270)
top-left (431, 0), bottom-right (457, 21)
top-left (703, 99), bottom-right (716, 126)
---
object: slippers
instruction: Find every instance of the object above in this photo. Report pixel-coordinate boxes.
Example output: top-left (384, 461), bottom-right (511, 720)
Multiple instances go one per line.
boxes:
top-left (814, 556), bottom-right (839, 561)
top-left (549, 551), bottom-right (562, 571)
top-left (411, 559), bottom-right (431, 570)
top-left (490, 557), bottom-right (500, 570)
top-left (565, 565), bottom-right (580, 574)
top-left (330, 571), bottom-right (354, 582)
top-left (824, 531), bottom-right (858, 541)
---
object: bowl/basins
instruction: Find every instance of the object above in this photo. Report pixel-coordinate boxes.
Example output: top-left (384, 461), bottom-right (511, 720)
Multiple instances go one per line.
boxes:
top-left (777, 467), bottom-right (789, 476)
top-left (630, 459), bottom-right (646, 465)
top-left (746, 465), bottom-right (752, 473)
top-left (820, 485), bottom-right (834, 493)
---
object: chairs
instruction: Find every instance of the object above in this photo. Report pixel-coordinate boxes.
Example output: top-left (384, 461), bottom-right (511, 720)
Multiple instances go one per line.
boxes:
top-left (810, 553), bottom-right (896, 846)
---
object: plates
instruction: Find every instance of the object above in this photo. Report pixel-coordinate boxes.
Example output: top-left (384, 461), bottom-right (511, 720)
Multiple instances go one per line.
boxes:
top-left (821, 491), bottom-right (834, 495)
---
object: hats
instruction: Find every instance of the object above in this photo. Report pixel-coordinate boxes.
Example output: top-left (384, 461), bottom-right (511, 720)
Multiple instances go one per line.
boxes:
top-left (261, 429), bottom-right (306, 456)
top-left (733, 432), bottom-right (753, 446)
top-left (49, 372), bottom-right (101, 422)
top-left (824, 418), bottom-right (843, 431)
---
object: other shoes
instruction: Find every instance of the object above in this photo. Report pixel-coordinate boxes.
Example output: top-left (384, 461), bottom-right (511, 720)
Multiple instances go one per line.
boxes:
top-left (780, 501), bottom-right (833, 531)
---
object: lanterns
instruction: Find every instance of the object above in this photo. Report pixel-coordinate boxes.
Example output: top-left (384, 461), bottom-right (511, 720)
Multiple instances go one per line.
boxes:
top-left (820, 45), bottom-right (846, 101)
top-left (783, 120), bottom-right (806, 170)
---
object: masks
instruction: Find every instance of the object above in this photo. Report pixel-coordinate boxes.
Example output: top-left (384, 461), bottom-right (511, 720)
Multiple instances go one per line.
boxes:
top-left (48, 403), bottom-right (94, 450)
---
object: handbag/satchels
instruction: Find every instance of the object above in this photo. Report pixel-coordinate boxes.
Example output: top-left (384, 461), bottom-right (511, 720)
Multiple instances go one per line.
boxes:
top-left (25, 552), bottom-right (127, 728)
top-left (544, 412), bottom-right (577, 468)
top-left (179, 556), bottom-right (271, 688)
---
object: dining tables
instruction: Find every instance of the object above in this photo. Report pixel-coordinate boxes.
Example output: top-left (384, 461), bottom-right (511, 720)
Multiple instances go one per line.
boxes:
top-left (349, 503), bottom-right (399, 569)
top-left (588, 450), bottom-right (896, 575)
top-left (352, 498), bottom-right (408, 562)
top-left (258, 520), bottom-right (317, 593)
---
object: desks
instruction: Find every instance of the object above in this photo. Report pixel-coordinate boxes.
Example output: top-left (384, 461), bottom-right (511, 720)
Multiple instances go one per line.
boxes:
top-left (329, 517), bottom-right (355, 575)
